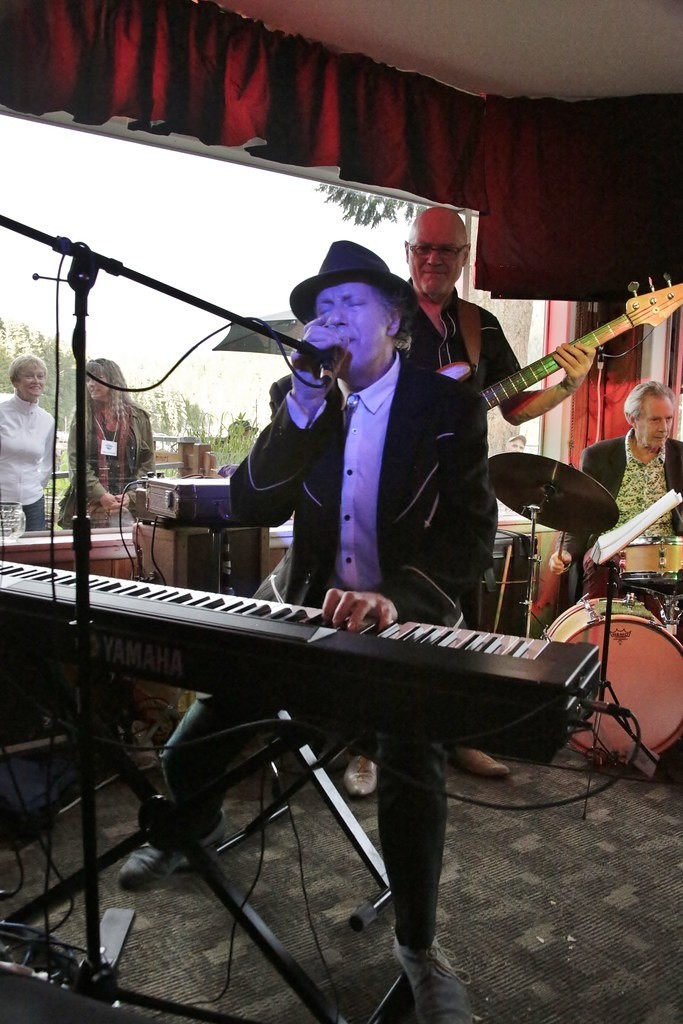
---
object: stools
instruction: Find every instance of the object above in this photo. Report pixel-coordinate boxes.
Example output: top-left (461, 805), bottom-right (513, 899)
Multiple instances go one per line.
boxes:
top-left (190, 696), bottom-right (394, 929)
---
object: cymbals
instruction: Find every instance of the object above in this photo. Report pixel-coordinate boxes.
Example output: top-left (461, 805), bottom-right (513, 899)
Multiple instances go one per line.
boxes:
top-left (489, 451), bottom-right (622, 537)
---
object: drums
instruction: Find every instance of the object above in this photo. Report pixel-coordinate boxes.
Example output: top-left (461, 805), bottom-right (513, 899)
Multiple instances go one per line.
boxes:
top-left (539, 597), bottom-right (683, 764)
top-left (614, 534), bottom-right (683, 583)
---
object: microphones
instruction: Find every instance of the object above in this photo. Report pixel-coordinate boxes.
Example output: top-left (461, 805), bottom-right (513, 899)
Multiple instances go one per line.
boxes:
top-left (320, 324), bottom-right (337, 382)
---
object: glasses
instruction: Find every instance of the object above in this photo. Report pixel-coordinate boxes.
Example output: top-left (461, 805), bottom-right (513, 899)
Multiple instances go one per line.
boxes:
top-left (408, 242), bottom-right (468, 261)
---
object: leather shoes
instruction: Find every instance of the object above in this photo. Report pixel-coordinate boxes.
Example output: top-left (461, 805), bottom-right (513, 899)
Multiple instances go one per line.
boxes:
top-left (446, 744), bottom-right (511, 779)
top-left (343, 754), bottom-right (378, 798)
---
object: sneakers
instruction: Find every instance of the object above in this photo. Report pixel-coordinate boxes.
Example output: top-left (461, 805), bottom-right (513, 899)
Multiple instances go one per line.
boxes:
top-left (117, 804), bottom-right (227, 892)
top-left (393, 925), bottom-right (474, 1024)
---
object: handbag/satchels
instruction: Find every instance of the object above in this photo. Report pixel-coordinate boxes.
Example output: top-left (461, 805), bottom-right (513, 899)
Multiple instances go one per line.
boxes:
top-left (56, 482), bottom-right (78, 530)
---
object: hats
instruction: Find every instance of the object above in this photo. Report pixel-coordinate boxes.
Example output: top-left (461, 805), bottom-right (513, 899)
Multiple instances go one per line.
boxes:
top-left (289, 240), bottom-right (419, 324)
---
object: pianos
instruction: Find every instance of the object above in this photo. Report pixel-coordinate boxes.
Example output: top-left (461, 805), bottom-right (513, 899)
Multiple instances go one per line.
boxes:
top-left (0, 558), bottom-right (603, 1024)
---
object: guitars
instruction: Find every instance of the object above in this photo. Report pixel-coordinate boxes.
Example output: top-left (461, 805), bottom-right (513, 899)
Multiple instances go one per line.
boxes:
top-left (433, 270), bottom-right (682, 413)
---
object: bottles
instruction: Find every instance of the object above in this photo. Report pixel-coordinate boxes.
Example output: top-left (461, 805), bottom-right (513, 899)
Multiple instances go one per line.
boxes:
top-left (1, 500), bottom-right (27, 546)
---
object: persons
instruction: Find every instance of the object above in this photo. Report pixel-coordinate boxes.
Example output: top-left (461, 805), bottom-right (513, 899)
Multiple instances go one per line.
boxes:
top-left (502, 435), bottom-right (527, 517)
top-left (343, 207), bottom-right (596, 798)
top-left (67, 358), bottom-right (156, 528)
top-left (549, 381), bottom-right (683, 645)
top-left (118, 240), bottom-right (499, 1024)
top-left (0, 354), bottom-right (55, 532)
